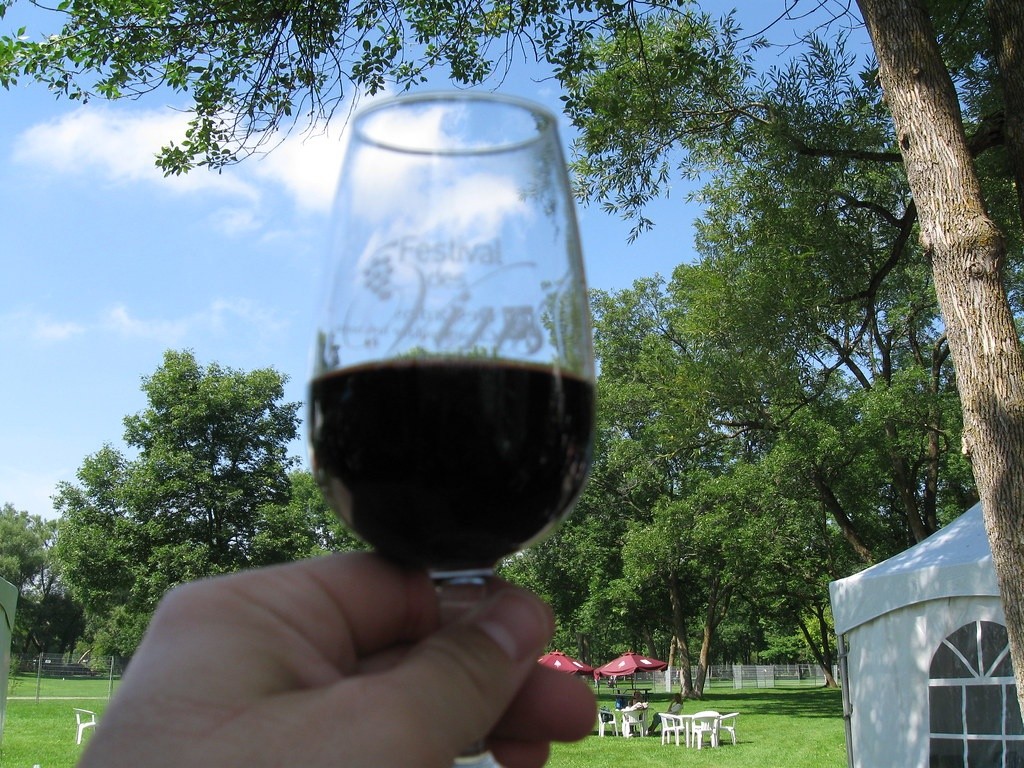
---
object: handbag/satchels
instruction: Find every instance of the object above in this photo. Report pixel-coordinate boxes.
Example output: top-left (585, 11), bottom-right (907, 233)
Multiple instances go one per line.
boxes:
top-left (601, 706), bottom-right (613, 721)
top-left (616, 698), bottom-right (626, 709)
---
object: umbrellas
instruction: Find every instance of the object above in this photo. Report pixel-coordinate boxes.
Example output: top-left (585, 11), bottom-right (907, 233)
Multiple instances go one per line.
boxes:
top-left (594, 650), bottom-right (669, 692)
top-left (537, 649), bottom-right (594, 677)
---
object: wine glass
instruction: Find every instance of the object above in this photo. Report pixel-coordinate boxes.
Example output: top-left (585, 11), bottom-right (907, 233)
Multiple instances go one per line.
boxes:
top-left (305, 92), bottom-right (599, 768)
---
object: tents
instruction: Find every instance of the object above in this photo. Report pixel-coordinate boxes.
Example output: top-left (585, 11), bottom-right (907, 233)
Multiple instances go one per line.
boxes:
top-left (0, 576), bottom-right (18, 745)
top-left (828, 499), bottom-right (1024, 768)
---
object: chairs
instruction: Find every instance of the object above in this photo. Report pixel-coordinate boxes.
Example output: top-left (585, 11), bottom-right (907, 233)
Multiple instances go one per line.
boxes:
top-left (73, 707), bottom-right (97, 745)
top-left (596, 707), bottom-right (740, 750)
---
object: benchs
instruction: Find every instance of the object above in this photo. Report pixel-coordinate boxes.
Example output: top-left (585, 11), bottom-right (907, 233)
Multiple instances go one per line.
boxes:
top-left (610, 693), bottom-right (654, 698)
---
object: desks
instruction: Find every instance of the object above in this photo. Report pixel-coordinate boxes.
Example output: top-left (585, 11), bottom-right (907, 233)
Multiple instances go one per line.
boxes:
top-left (615, 708), bottom-right (654, 738)
top-left (614, 688), bottom-right (652, 703)
top-left (675, 715), bottom-right (721, 749)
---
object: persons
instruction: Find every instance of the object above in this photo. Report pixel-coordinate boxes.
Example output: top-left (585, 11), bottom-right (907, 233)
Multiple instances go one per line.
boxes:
top-left (74, 552), bottom-right (599, 768)
top-left (648, 693), bottom-right (683, 736)
